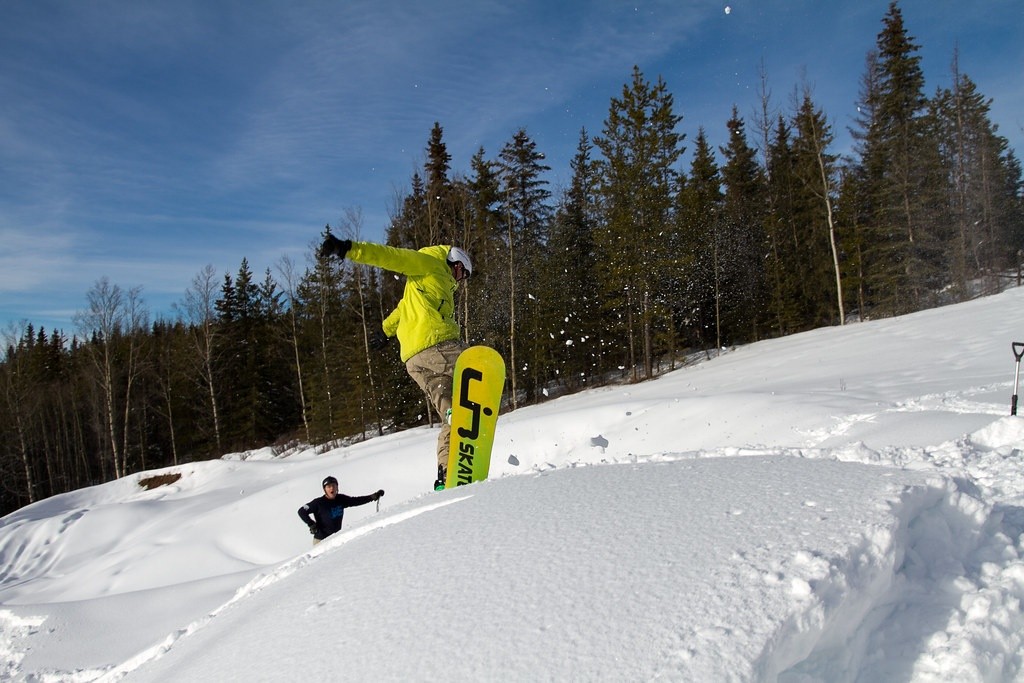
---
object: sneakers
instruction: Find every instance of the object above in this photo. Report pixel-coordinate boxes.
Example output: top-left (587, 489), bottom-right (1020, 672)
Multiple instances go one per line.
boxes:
top-left (445, 408), bottom-right (452, 426)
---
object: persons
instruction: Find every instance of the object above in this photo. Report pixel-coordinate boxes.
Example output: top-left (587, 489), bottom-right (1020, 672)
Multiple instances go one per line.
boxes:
top-left (297, 476), bottom-right (385, 548)
top-left (315, 230), bottom-right (474, 484)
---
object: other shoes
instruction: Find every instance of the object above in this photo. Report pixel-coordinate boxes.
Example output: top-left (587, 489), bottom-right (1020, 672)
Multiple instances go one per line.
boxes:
top-left (433, 464), bottom-right (447, 491)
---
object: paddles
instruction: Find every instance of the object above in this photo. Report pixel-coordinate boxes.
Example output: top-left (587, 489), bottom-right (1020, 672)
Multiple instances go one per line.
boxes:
top-left (447, 345), bottom-right (506, 493)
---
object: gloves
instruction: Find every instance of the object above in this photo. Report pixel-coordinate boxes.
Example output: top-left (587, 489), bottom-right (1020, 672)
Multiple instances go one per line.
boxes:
top-left (309, 523), bottom-right (319, 534)
top-left (371, 490), bottom-right (384, 501)
top-left (320, 235), bottom-right (352, 261)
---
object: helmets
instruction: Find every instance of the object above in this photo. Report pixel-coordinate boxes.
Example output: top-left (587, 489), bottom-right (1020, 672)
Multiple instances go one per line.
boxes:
top-left (323, 477), bottom-right (338, 490)
top-left (447, 246), bottom-right (472, 279)
